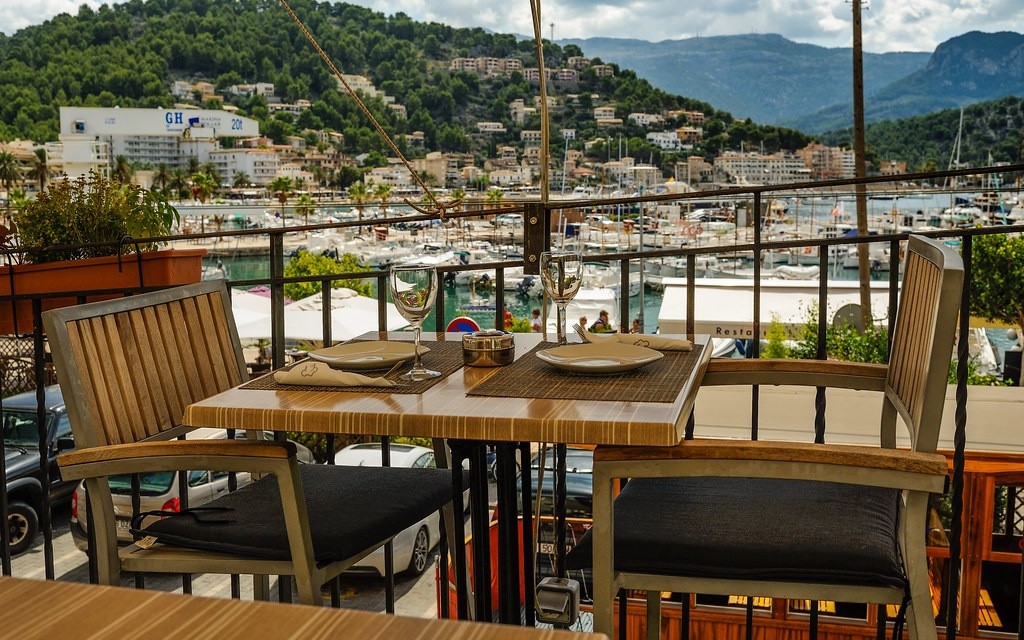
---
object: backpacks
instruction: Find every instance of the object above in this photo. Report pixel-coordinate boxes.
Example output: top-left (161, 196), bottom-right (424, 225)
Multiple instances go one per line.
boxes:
top-left (588, 321), bottom-right (601, 333)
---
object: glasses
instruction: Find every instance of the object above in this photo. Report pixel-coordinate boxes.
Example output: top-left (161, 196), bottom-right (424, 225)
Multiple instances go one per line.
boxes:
top-left (583, 319), bottom-right (587, 321)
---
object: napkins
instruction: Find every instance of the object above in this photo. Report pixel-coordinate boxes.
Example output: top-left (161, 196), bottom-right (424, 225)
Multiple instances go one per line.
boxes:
top-left (274, 361), bottom-right (396, 387)
top-left (582, 328), bottom-right (693, 351)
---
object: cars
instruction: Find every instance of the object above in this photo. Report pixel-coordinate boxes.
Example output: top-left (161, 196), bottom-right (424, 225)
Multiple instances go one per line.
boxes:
top-left (485, 446), bottom-right (599, 603)
top-left (67, 428), bottom-right (317, 558)
top-left (324, 442), bottom-right (470, 589)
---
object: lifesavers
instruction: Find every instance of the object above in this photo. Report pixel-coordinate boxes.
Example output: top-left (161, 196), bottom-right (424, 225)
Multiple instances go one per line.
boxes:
top-left (683, 226), bottom-right (703, 236)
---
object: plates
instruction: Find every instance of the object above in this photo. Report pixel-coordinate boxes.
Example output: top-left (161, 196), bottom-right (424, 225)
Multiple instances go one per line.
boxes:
top-left (308, 341), bottom-right (431, 370)
top-left (536, 343), bottom-right (664, 375)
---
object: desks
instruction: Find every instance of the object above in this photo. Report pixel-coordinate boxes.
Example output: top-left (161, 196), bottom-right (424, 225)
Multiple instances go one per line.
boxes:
top-left (183, 331), bottom-right (713, 627)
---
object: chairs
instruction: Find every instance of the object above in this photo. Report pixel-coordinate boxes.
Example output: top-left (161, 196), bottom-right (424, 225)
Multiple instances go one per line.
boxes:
top-left (41, 279), bottom-right (470, 608)
top-left (563, 234), bottom-right (965, 640)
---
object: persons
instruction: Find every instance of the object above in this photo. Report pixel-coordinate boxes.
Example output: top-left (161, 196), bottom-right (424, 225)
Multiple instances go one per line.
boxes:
top-left (529, 309), bottom-right (543, 332)
top-left (494, 303), bottom-right (513, 329)
top-left (592, 310), bottom-right (612, 334)
top-left (629, 319), bottom-right (639, 334)
top-left (579, 316), bottom-right (587, 332)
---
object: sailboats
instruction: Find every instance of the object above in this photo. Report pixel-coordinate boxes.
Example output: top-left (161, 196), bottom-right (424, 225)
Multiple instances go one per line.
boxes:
top-left (185, 106), bottom-right (1020, 302)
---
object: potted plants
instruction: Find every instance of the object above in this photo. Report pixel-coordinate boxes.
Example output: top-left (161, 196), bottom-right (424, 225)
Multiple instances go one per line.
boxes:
top-left (0, 168), bottom-right (207, 337)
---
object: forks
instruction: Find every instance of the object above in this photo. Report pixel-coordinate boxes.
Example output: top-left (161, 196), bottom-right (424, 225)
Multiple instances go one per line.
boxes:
top-left (383, 360), bottom-right (406, 379)
top-left (572, 323), bottom-right (586, 337)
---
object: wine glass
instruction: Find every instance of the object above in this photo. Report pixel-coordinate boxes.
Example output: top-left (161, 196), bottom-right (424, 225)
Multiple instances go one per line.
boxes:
top-left (389, 265), bottom-right (442, 381)
top-left (539, 251), bottom-right (583, 346)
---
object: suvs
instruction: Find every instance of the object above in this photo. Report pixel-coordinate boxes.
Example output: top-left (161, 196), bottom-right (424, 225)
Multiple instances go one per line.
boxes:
top-left (0, 382), bottom-right (85, 558)
